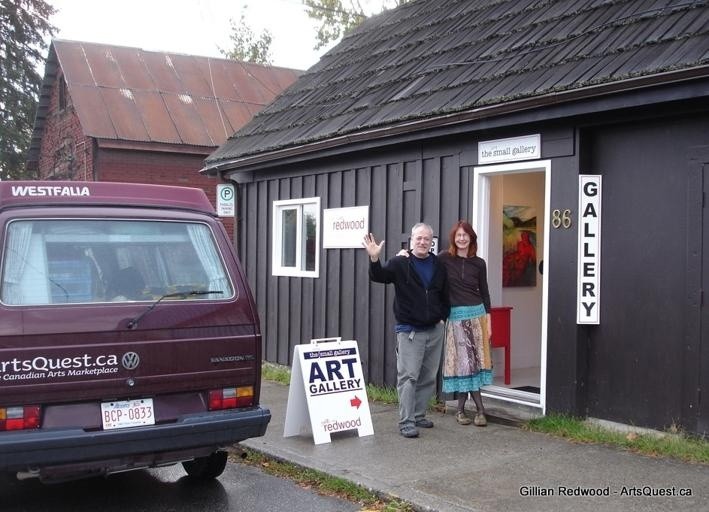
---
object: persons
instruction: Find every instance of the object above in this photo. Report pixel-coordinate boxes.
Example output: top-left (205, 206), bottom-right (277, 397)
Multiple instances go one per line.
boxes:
top-left (436, 218), bottom-right (495, 427)
top-left (360, 220), bottom-right (450, 439)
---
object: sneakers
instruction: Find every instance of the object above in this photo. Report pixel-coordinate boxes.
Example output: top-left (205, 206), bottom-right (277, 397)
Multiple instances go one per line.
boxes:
top-left (416, 420), bottom-right (433, 427)
top-left (402, 426), bottom-right (418, 437)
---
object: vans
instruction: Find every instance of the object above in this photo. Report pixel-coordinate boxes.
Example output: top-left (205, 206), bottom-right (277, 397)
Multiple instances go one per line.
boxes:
top-left (0, 179), bottom-right (271, 484)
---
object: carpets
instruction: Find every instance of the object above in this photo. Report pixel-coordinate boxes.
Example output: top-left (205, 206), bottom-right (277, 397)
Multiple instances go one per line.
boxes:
top-left (510, 385), bottom-right (540, 395)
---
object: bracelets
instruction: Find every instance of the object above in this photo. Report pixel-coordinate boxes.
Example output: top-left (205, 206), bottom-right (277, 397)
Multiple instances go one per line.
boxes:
top-left (370, 255), bottom-right (378, 260)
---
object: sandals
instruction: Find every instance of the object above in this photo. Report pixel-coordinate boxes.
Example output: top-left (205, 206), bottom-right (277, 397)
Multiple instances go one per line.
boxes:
top-left (479, 413), bottom-right (487, 425)
top-left (456, 411), bottom-right (470, 424)
